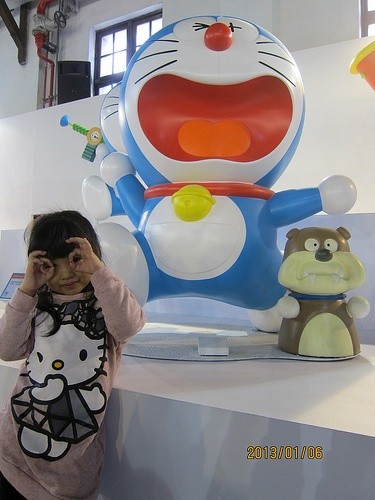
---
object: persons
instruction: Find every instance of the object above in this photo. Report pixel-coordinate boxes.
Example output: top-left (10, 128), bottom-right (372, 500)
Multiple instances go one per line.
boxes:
top-left (0, 210), bottom-right (147, 500)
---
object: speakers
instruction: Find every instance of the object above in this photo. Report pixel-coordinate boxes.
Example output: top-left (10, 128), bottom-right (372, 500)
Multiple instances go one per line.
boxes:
top-left (57, 61), bottom-right (91, 105)
